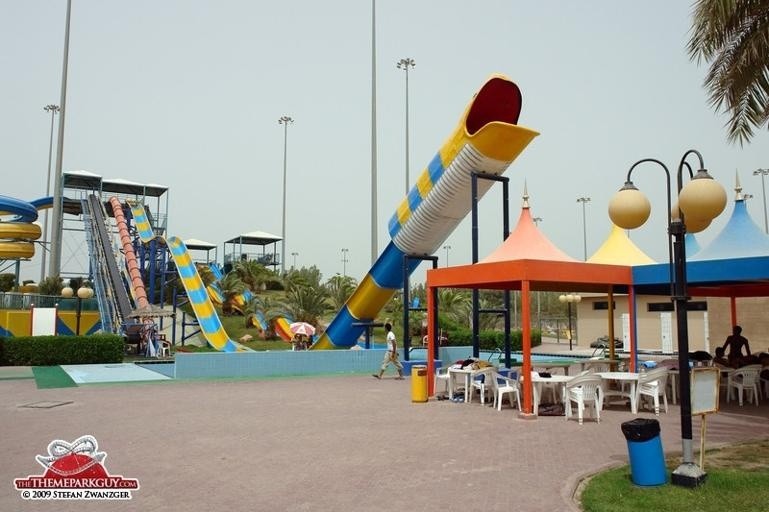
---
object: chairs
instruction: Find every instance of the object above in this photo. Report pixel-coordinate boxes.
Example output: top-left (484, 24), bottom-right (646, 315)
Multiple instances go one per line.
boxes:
top-left (434, 355), bottom-right (769, 425)
top-left (157, 340), bottom-right (171, 358)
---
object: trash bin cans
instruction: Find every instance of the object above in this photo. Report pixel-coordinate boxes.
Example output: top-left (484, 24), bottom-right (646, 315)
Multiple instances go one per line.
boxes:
top-left (411, 365), bottom-right (429, 403)
top-left (621, 417), bottom-right (669, 486)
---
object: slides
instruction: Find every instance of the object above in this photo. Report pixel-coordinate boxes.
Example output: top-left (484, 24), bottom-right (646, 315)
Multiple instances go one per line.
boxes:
top-left (1, 195), bottom-right (71, 259)
top-left (125, 74), bottom-right (540, 352)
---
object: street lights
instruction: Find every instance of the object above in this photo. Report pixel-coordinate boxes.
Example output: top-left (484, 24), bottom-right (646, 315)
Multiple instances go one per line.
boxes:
top-left (291, 252), bottom-right (299, 270)
top-left (752, 168), bottom-right (769, 234)
top-left (278, 116), bottom-right (295, 273)
top-left (41, 104), bottom-right (61, 282)
top-left (576, 197), bottom-right (592, 261)
top-left (340, 247), bottom-right (349, 277)
top-left (608, 149), bottom-right (728, 490)
top-left (397, 57), bottom-right (416, 196)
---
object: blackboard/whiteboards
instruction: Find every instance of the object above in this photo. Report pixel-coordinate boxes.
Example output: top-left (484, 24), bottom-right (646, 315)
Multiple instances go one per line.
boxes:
top-left (31, 308), bottom-right (55, 337)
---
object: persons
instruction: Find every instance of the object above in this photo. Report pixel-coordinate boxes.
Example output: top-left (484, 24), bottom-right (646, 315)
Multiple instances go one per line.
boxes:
top-left (714, 347), bottom-right (736, 378)
top-left (292, 333), bottom-right (313, 351)
top-left (722, 324), bottom-right (751, 367)
top-left (371, 323), bottom-right (406, 381)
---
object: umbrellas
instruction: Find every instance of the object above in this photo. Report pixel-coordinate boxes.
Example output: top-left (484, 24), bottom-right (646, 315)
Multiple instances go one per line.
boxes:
top-left (289, 321), bottom-right (316, 336)
